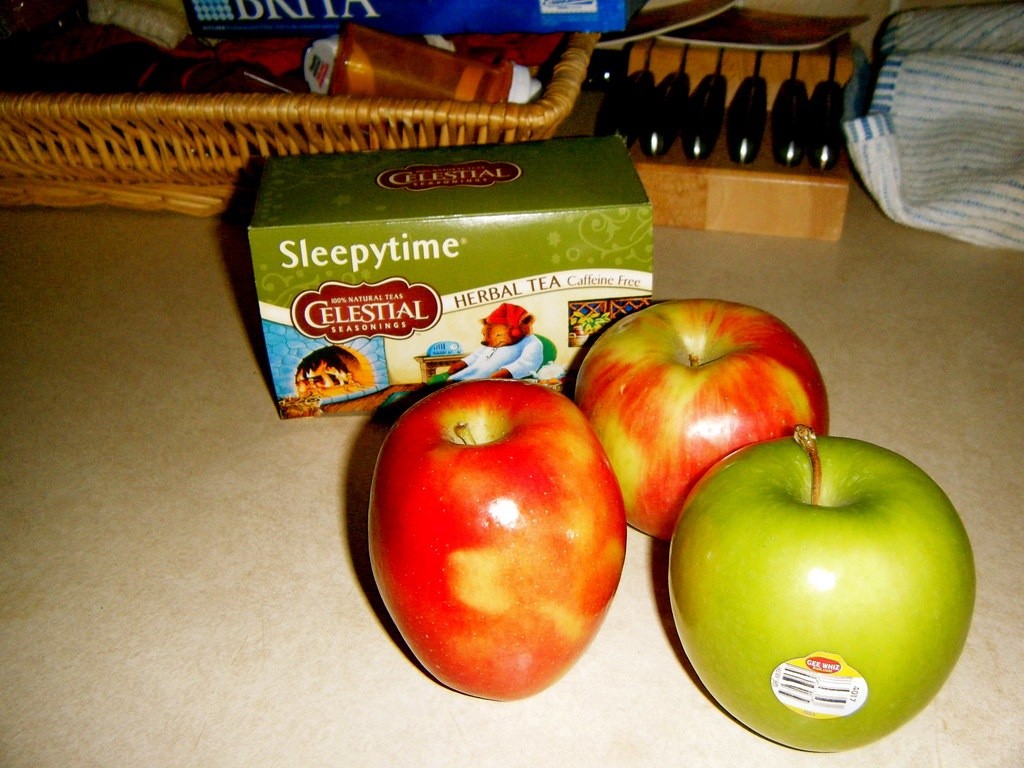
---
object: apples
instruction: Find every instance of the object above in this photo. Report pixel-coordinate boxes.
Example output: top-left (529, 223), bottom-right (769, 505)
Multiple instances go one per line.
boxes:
top-left (574, 297), bottom-right (830, 544)
top-left (670, 423), bottom-right (978, 754)
top-left (368, 377), bottom-right (627, 703)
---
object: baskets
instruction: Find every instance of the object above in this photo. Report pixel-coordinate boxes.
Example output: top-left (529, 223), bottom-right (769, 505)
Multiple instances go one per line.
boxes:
top-left (0, 32), bottom-right (605, 219)
